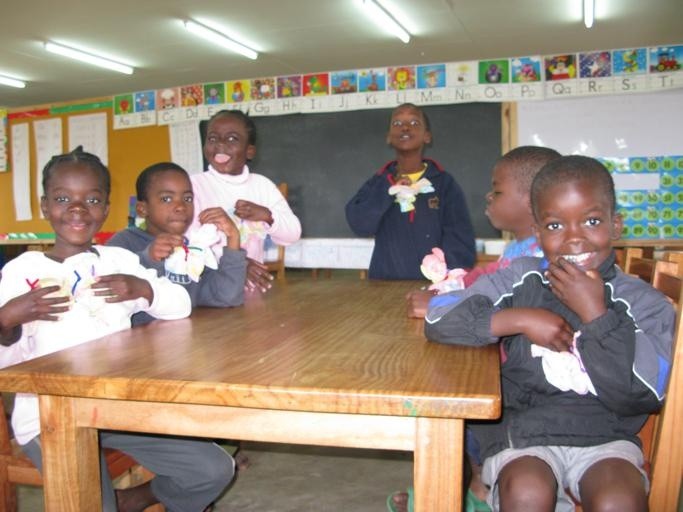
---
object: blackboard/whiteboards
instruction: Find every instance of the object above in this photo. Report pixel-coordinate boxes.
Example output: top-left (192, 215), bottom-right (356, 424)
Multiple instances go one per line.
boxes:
top-left (199, 100), bottom-right (510, 240)
top-left (511, 87), bottom-right (683, 247)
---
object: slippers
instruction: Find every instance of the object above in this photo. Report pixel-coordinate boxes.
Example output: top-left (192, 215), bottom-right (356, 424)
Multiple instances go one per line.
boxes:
top-left (387, 489), bottom-right (477, 512)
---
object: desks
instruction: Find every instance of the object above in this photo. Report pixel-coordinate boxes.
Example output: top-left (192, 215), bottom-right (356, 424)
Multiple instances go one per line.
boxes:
top-left (0, 272), bottom-right (506, 510)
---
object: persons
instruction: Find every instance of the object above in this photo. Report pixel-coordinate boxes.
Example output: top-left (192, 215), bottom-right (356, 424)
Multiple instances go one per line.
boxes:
top-left (182, 106), bottom-right (303, 299)
top-left (381, 139), bottom-right (563, 511)
top-left (340, 100), bottom-right (479, 284)
top-left (0, 143), bottom-right (237, 511)
top-left (420, 154), bottom-right (680, 512)
top-left (102, 160), bottom-right (250, 474)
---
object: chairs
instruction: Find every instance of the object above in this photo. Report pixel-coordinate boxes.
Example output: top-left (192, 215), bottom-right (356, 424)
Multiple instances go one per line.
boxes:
top-left (621, 247), bottom-right (683, 511)
